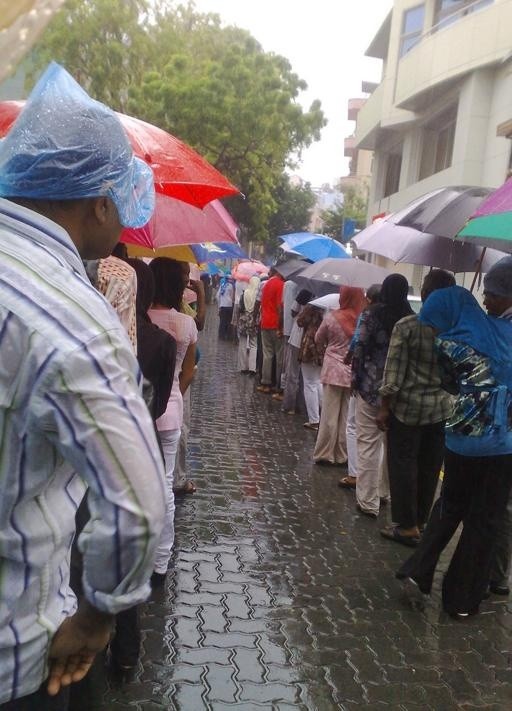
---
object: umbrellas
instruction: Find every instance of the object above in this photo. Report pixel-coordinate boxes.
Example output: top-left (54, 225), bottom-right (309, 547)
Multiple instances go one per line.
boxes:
top-left (232, 262), bottom-right (268, 280)
top-left (383, 183), bottom-right (498, 292)
top-left (270, 259), bottom-right (312, 282)
top-left (198, 263), bottom-right (209, 270)
top-left (149, 192), bottom-right (240, 251)
top-left (278, 231), bottom-right (352, 263)
top-left (453, 175), bottom-right (512, 257)
top-left (279, 242), bottom-right (302, 257)
top-left (192, 241), bottom-right (245, 263)
top-left (126, 245), bottom-right (198, 262)
top-left (351, 213), bottom-right (507, 274)
top-left (120, 219), bottom-right (156, 251)
top-left (2, 99), bottom-right (245, 212)
top-left (296, 256), bottom-right (391, 292)
top-left (208, 262), bottom-right (221, 274)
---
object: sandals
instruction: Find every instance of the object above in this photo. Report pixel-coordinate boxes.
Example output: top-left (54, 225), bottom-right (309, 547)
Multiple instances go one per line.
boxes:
top-left (490, 583), bottom-right (509, 594)
top-left (281, 408), bottom-right (295, 415)
top-left (305, 422), bottom-right (318, 430)
top-left (272, 394), bottom-right (284, 400)
top-left (481, 585), bottom-right (490, 599)
top-left (256, 386), bottom-right (272, 393)
top-left (173, 480), bottom-right (194, 493)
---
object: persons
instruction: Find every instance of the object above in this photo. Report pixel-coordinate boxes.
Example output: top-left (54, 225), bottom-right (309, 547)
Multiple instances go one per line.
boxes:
top-left (279, 289), bottom-right (318, 415)
top-left (256, 271), bottom-right (285, 393)
top-left (313, 287), bottom-right (370, 466)
top-left (0, 62), bottom-right (174, 711)
top-left (374, 268), bottom-right (456, 548)
top-left (341, 284), bottom-right (384, 487)
top-left (71, 254), bottom-right (142, 680)
top-left (208, 275), bottom-right (219, 304)
top-left (236, 276), bottom-right (262, 375)
top-left (176, 260), bottom-right (205, 370)
top-left (234, 280), bottom-right (247, 326)
top-left (148, 256), bottom-right (201, 582)
top-left (218, 274), bottom-right (235, 340)
top-left (253, 268), bottom-right (276, 373)
top-left (296, 303), bottom-right (327, 431)
top-left (395, 286), bottom-right (512, 618)
top-left (274, 280), bottom-right (301, 400)
top-left (127, 260), bottom-right (179, 473)
top-left (349, 274), bottom-right (418, 516)
top-left (482, 255), bottom-right (512, 596)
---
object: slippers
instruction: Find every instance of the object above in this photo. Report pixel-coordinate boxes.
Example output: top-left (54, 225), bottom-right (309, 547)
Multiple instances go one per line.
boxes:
top-left (383, 527), bottom-right (421, 546)
top-left (340, 477), bottom-right (357, 490)
